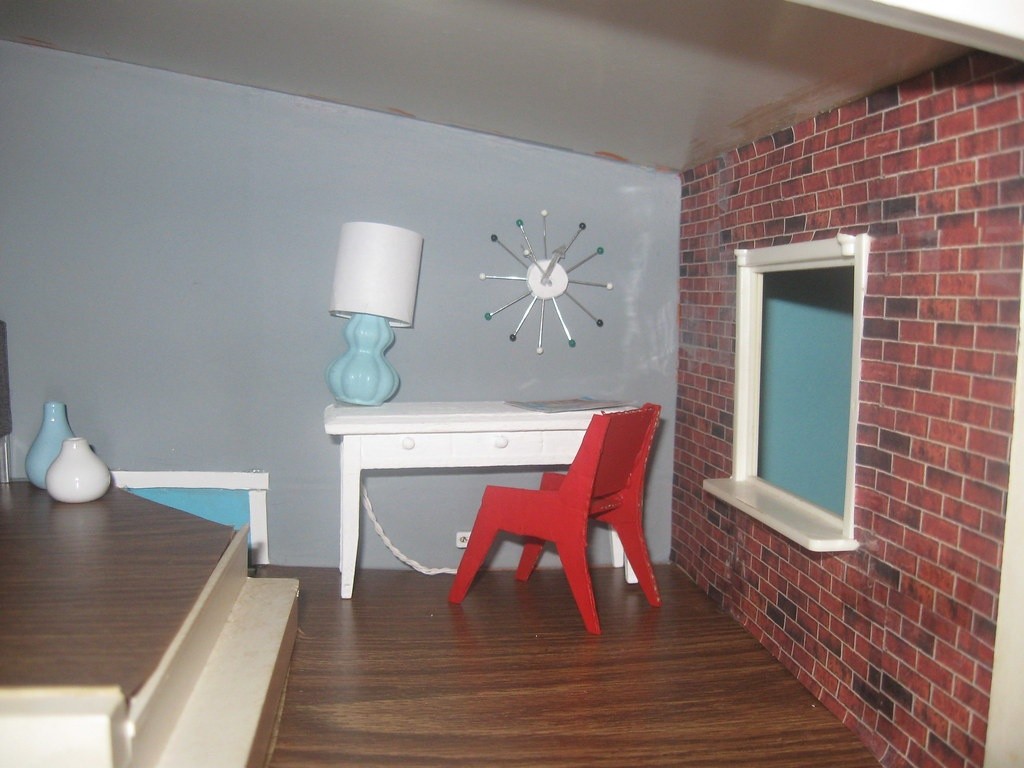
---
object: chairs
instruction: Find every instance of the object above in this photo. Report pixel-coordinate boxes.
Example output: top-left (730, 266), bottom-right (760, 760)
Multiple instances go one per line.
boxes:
top-left (448, 402), bottom-right (662, 634)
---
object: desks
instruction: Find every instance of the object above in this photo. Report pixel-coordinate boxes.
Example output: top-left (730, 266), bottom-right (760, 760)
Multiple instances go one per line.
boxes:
top-left (324, 404), bottom-right (639, 599)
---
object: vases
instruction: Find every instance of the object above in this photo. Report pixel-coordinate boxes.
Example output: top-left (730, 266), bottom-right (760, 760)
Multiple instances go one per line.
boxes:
top-left (45, 437), bottom-right (111, 504)
top-left (25, 401), bottom-right (75, 489)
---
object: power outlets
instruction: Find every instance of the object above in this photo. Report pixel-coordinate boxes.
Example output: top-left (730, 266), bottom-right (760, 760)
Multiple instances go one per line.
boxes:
top-left (455, 532), bottom-right (472, 548)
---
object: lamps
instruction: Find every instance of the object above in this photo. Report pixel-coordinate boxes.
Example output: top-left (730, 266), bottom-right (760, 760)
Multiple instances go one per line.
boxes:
top-left (325, 222), bottom-right (423, 407)
